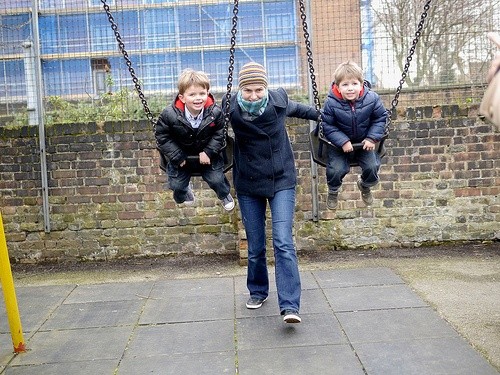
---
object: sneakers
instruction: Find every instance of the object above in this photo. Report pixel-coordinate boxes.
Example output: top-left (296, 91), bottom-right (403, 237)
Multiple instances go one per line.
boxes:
top-left (221, 194), bottom-right (235, 211)
top-left (357, 179), bottom-right (373, 205)
top-left (327, 195), bottom-right (338, 210)
top-left (283, 309), bottom-right (301, 323)
top-left (246, 296), bottom-right (269, 308)
top-left (184, 190), bottom-right (195, 206)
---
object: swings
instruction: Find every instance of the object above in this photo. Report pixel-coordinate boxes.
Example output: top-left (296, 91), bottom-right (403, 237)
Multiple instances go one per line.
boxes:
top-left (99, 0), bottom-right (239, 177)
top-left (298, 0), bottom-right (431, 168)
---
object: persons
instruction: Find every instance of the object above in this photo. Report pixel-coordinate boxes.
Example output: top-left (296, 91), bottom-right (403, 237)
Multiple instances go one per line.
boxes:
top-left (221, 62), bottom-right (324, 323)
top-left (316, 61), bottom-right (387, 210)
top-left (154, 68), bottom-right (235, 211)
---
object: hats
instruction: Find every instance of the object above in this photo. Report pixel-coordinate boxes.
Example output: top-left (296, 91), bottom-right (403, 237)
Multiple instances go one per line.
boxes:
top-left (238, 61), bottom-right (268, 89)
